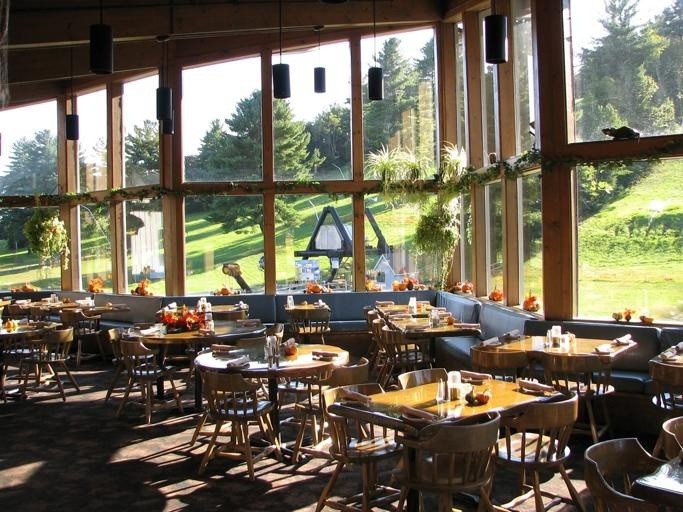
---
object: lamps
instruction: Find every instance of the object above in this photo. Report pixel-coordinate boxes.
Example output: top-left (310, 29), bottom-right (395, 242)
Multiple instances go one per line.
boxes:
top-left (89, 2), bottom-right (113, 75)
top-left (367, 2), bottom-right (384, 101)
top-left (156, 35), bottom-right (173, 121)
top-left (272, 2), bottom-right (291, 100)
top-left (484, 1), bottom-right (509, 65)
top-left (162, 43), bottom-right (176, 135)
top-left (65, 46), bottom-right (80, 141)
top-left (314, 25), bottom-right (326, 94)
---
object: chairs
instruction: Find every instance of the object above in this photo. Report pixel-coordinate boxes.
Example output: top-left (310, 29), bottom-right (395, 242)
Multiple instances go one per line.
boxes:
top-left (0, 279), bottom-right (683, 512)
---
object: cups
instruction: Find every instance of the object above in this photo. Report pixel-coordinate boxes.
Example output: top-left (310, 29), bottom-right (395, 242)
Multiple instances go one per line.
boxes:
top-left (447, 370), bottom-right (461, 400)
top-left (268, 336), bottom-right (277, 356)
top-left (552, 325), bottom-right (561, 349)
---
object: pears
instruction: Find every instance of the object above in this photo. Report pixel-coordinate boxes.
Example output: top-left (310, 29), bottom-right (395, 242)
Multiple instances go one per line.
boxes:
top-left (285, 344), bottom-right (297, 356)
top-left (466, 386), bottom-right (476, 404)
top-left (447, 317), bottom-right (454, 324)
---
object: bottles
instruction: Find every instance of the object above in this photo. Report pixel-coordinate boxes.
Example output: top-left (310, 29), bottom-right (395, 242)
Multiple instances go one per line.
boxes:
top-left (0, 294), bottom-right (441, 333)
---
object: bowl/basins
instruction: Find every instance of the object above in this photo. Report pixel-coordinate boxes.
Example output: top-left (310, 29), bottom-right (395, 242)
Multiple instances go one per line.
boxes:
top-left (641, 317), bottom-right (653, 323)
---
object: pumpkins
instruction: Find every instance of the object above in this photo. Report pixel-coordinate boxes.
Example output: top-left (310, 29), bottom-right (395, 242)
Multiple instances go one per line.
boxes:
top-left (476, 388), bottom-right (490, 404)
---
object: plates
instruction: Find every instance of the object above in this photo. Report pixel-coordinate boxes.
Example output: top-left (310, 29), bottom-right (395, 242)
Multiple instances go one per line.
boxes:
top-left (338, 377), bottom-right (548, 423)
top-left (416, 299), bottom-right (481, 332)
top-left (658, 352), bottom-right (680, 360)
top-left (485, 332), bottom-right (634, 355)
